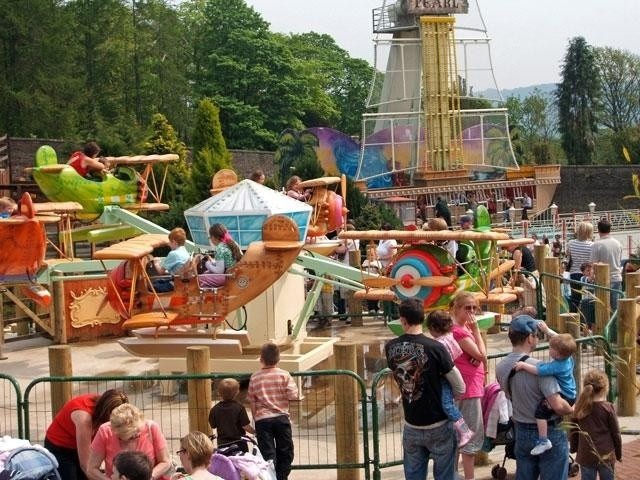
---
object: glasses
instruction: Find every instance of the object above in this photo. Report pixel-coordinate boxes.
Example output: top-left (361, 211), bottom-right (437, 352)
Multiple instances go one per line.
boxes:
top-left (464, 305), bottom-right (478, 310)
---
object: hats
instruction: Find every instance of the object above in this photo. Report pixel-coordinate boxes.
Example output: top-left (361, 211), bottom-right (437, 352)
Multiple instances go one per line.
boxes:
top-left (456, 216), bottom-right (473, 224)
top-left (509, 315), bottom-right (543, 332)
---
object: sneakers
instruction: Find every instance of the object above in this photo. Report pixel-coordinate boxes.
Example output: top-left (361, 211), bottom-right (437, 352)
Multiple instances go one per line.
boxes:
top-left (370, 309), bottom-right (376, 314)
top-left (530, 439), bottom-right (552, 455)
top-left (339, 317), bottom-right (351, 324)
top-left (313, 318), bottom-right (320, 321)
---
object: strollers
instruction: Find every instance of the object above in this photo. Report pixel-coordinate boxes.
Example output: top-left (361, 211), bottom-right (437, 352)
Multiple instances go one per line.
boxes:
top-left (492, 415), bottom-right (579, 480)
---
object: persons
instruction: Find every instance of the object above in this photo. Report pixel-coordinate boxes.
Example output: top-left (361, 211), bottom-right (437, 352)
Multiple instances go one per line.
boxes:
top-left (0, 196), bottom-right (15, 219)
top-left (21, 182), bottom-right (42, 201)
top-left (207, 376), bottom-right (257, 459)
top-left (198, 223), bottom-right (244, 287)
top-left (426, 309), bottom-right (476, 449)
top-left (512, 321), bottom-right (578, 455)
top-left (497, 313), bottom-right (576, 479)
top-left (85, 404), bottom-right (171, 480)
top-left (66, 142), bottom-right (110, 181)
top-left (110, 450), bottom-right (153, 479)
top-left (169, 429), bottom-right (226, 480)
top-left (307, 180), bottom-right (640, 340)
top-left (247, 344), bottom-right (304, 480)
top-left (43, 389), bottom-right (129, 480)
top-left (569, 368), bottom-right (624, 480)
top-left (448, 288), bottom-right (491, 479)
top-left (384, 295), bottom-right (468, 478)
top-left (249, 170), bottom-right (310, 199)
top-left (148, 227), bottom-right (190, 294)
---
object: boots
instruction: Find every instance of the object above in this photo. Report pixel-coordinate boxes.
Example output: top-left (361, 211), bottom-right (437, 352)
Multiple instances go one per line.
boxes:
top-left (455, 417), bottom-right (477, 449)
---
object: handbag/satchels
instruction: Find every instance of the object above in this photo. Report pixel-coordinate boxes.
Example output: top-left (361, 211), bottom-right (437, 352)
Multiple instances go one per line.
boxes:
top-left (564, 256), bottom-right (572, 272)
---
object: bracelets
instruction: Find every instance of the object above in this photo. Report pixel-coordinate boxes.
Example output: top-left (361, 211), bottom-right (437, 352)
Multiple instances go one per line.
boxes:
top-left (483, 370), bottom-right (489, 376)
